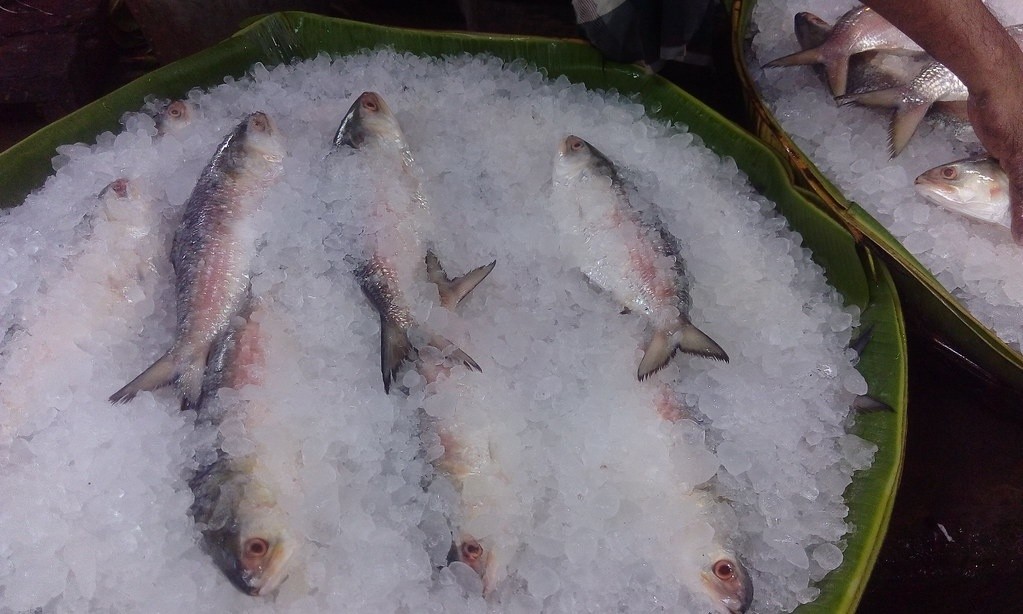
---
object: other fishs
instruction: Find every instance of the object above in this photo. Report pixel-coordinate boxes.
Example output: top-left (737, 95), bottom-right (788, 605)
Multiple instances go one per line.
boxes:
top-left (0, 1), bottom-right (1021, 613)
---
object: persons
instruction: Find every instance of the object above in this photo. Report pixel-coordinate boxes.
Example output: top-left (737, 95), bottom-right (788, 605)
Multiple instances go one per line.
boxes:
top-left (465, 0), bottom-right (1023, 245)
